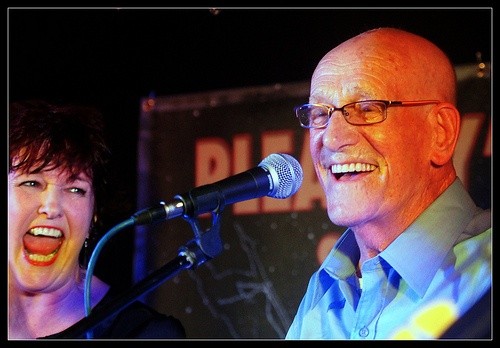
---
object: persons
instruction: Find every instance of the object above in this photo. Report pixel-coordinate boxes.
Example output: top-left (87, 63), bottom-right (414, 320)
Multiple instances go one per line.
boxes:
top-left (282, 27), bottom-right (491, 339)
top-left (10, 98), bottom-right (188, 341)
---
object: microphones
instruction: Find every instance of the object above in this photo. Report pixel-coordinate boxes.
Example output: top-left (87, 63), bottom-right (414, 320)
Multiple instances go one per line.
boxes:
top-left (133, 153), bottom-right (303, 225)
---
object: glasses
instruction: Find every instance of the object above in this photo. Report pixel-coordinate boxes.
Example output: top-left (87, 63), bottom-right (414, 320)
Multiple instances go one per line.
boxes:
top-left (295, 93), bottom-right (438, 129)
